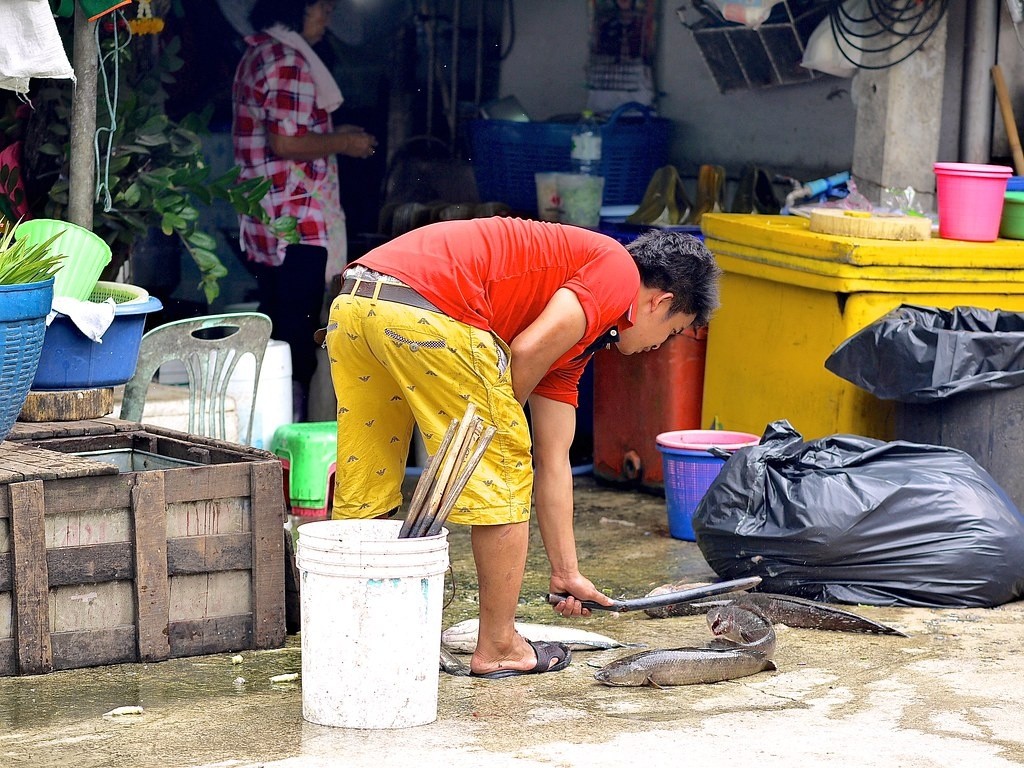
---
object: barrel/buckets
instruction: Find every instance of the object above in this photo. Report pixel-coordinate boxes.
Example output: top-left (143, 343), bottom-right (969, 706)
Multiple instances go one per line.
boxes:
top-left (932, 162), bottom-right (1013, 242)
top-left (295, 518), bottom-right (449, 730)
top-left (202, 338), bottom-right (292, 454)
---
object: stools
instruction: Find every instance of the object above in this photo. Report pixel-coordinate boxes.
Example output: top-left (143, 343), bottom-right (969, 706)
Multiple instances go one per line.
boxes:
top-left (272, 421), bottom-right (338, 518)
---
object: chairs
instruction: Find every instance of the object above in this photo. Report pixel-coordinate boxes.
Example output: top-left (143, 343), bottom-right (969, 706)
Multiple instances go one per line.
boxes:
top-left (118, 312), bottom-right (272, 447)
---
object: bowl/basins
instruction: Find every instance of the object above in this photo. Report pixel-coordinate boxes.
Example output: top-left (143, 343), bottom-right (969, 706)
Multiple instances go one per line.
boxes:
top-left (30, 295), bottom-right (163, 391)
top-left (999, 191), bottom-right (1024, 240)
top-left (478, 96), bottom-right (530, 124)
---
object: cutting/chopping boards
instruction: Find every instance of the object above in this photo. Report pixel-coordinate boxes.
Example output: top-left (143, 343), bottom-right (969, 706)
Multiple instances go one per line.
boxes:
top-left (811, 208), bottom-right (932, 241)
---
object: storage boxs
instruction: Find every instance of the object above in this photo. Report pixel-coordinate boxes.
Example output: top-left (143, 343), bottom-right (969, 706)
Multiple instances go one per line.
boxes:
top-left (105, 381), bottom-right (239, 445)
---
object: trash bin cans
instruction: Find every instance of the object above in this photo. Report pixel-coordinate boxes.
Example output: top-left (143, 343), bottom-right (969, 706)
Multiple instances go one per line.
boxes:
top-left (655, 429), bottom-right (763, 543)
top-left (895, 304), bottom-right (1023, 520)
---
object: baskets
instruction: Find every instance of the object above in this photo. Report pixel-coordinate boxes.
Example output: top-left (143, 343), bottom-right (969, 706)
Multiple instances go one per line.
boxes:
top-left (94, 282), bottom-right (147, 306)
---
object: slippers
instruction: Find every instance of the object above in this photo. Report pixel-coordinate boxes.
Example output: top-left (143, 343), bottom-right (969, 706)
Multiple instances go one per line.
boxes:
top-left (470, 637), bottom-right (573, 678)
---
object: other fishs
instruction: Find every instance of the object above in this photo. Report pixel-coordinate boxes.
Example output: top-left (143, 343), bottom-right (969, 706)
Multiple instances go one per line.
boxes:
top-left (595, 580), bottom-right (911, 691)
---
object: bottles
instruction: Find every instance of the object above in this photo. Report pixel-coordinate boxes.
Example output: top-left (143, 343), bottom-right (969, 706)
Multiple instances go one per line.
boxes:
top-left (571, 108), bottom-right (602, 174)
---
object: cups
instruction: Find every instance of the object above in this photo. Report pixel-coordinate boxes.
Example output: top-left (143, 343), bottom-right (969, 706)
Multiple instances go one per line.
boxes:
top-left (535, 173), bottom-right (569, 224)
top-left (558, 175), bottom-right (604, 227)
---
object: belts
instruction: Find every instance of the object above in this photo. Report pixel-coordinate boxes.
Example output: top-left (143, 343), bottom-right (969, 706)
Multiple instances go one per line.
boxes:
top-left (339, 280), bottom-right (444, 314)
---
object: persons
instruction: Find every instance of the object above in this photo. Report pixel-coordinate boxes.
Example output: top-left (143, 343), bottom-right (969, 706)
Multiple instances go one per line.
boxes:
top-left (231, 0), bottom-right (380, 422)
top-left (326, 216), bottom-right (720, 679)
